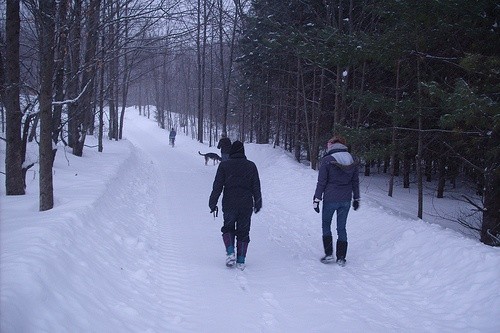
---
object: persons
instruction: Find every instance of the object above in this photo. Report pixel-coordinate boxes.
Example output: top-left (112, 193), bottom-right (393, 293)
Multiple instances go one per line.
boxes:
top-left (313, 137), bottom-right (360, 267)
top-left (169, 128), bottom-right (176, 147)
top-left (217, 131), bottom-right (231, 159)
top-left (209, 140), bottom-right (262, 270)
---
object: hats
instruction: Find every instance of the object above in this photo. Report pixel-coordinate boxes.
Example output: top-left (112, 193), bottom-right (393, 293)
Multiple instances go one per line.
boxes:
top-left (222, 132), bottom-right (226, 136)
top-left (229, 141), bottom-right (245, 155)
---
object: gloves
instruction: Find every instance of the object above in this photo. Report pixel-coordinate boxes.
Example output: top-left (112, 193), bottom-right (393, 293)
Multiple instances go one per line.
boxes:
top-left (210, 206), bottom-right (216, 213)
top-left (352, 197), bottom-right (360, 210)
top-left (254, 204), bottom-right (261, 214)
top-left (313, 196), bottom-right (322, 214)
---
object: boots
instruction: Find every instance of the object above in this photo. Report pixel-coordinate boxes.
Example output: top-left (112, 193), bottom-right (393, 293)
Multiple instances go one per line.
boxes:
top-left (336, 239), bottom-right (348, 267)
top-left (320, 235), bottom-right (334, 264)
top-left (223, 232), bottom-right (236, 267)
top-left (237, 241), bottom-right (248, 268)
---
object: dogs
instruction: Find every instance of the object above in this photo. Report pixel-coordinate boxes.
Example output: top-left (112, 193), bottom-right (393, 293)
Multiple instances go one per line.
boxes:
top-left (198, 151), bottom-right (222, 166)
